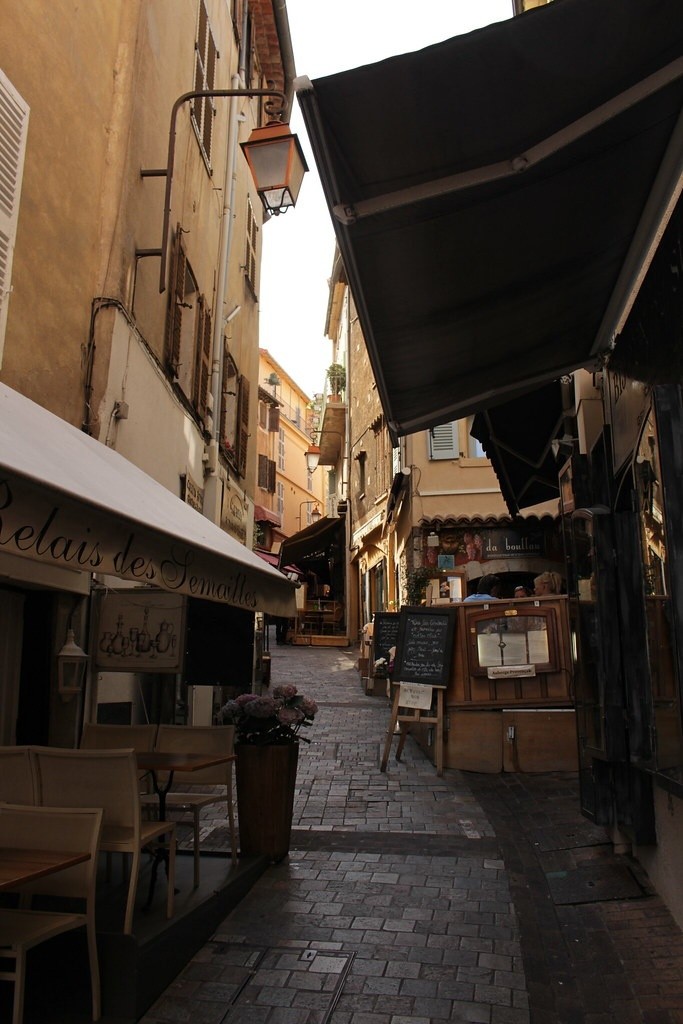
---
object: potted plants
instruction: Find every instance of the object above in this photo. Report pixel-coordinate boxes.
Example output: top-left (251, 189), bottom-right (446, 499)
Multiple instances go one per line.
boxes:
top-left (326, 363), bottom-right (346, 403)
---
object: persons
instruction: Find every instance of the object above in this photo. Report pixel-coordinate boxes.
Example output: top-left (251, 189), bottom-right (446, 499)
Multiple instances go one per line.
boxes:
top-left (533, 572), bottom-right (562, 597)
top-left (276, 623), bottom-right (290, 646)
top-left (463, 573), bottom-right (504, 602)
top-left (514, 586), bottom-right (535, 599)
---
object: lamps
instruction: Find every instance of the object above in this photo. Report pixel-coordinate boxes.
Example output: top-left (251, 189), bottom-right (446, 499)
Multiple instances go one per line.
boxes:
top-left (304, 438), bottom-right (321, 473)
top-left (311, 508), bottom-right (321, 523)
top-left (238, 110), bottom-right (310, 217)
top-left (56, 594), bottom-right (92, 704)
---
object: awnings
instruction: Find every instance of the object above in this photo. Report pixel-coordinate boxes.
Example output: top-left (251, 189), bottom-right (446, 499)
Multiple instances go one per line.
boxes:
top-left (0, 374), bottom-right (302, 620)
top-left (253, 504), bottom-right (282, 529)
top-left (293, 2), bottom-right (682, 436)
top-left (468, 380), bottom-right (562, 518)
top-left (252, 546), bottom-right (302, 573)
top-left (277, 513), bottom-right (345, 573)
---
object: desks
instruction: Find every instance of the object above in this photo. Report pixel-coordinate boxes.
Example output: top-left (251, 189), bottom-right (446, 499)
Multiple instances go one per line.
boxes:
top-left (304, 609), bottom-right (333, 634)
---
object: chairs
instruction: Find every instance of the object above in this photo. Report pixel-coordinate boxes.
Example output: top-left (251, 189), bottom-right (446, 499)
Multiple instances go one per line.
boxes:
top-left (321, 607), bottom-right (344, 635)
top-left (79, 721), bottom-right (158, 822)
top-left (0, 804), bottom-right (106, 1024)
top-left (154, 724), bottom-right (238, 921)
top-left (28, 747), bottom-right (178, 935)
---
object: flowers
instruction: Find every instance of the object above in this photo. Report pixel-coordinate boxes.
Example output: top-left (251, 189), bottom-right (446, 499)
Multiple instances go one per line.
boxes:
top-left (213, 684), bottom-right (319, 745)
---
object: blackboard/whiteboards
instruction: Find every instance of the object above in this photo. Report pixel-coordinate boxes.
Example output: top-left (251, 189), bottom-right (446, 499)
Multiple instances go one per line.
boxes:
top-left (372, 609), bottom-right (401, 648)
top-left (390, 604), bottom-right (458, 690)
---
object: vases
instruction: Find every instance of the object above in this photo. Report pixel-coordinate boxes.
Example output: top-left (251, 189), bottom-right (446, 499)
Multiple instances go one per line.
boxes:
top-left (235, 745), bottom-right (299, 866)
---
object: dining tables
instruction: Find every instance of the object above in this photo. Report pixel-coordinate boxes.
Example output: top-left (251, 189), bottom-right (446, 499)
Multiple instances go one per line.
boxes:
top-left (0, 848), bottom-right (91, 892)
top-left (138, 753), bottom-right (237, 915)
